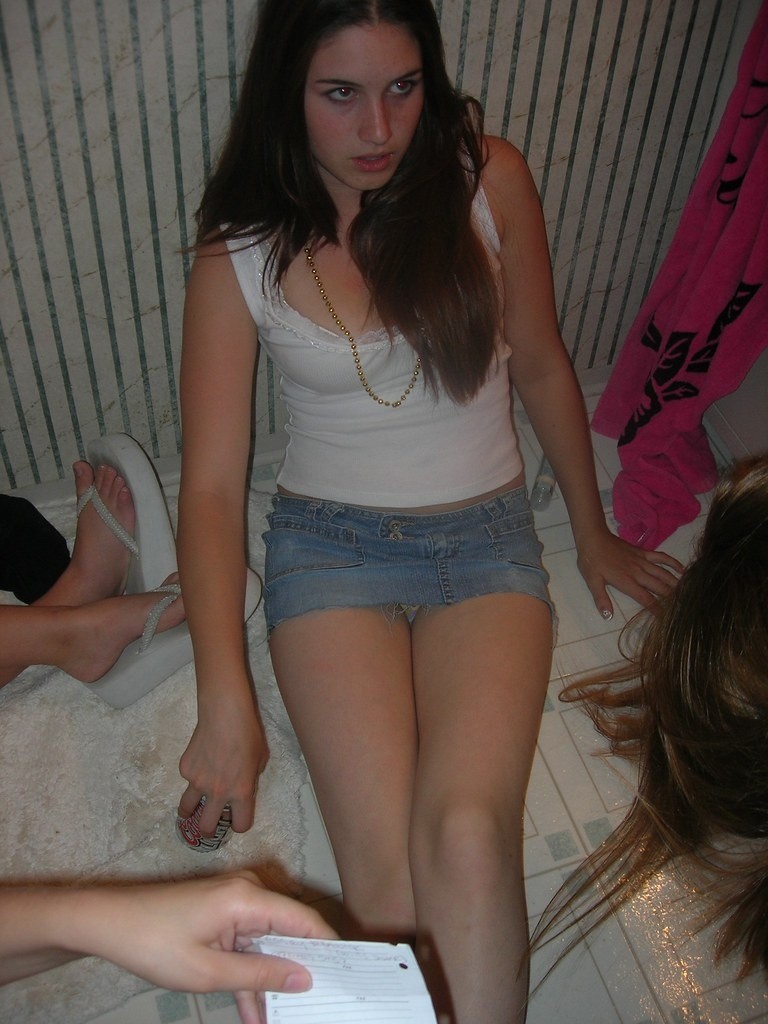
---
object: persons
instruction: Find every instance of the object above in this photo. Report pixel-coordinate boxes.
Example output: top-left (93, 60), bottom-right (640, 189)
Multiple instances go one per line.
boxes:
top-left (0, 869), bottom-right (341, 1024)
top-left (1, 434), bottom-right (261, 709)
top-left (178, 1), bottom-right (682, 1024)
top-left (515, 452), bottom-right (768, 1024)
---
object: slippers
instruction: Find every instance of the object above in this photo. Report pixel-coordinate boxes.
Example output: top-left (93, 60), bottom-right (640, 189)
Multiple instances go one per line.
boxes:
top-left (71, 433), bottom-right (179, 596)
top-left (85, 549), bottom-right (263, 713)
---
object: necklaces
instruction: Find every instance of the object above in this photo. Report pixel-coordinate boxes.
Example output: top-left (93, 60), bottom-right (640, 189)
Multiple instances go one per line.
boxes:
top-left (302, 240), bottom-right (421, 407)
